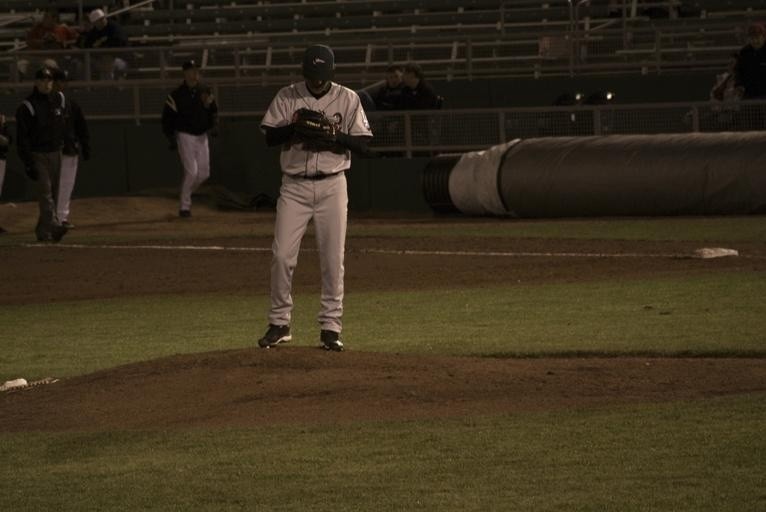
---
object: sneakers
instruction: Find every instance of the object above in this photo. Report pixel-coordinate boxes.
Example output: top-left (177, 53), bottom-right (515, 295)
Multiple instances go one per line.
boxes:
top-left (179, 210), bottom-right (192, 219)
top-left (319, 330), bottom-right (344, 352)
top-left (58, 220), bottom-right (75, 229)
top-left (257, 323), bottom-right (293, 348)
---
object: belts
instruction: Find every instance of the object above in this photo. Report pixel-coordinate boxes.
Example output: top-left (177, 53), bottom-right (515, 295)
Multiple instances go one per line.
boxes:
top-left (304, 171), bottom-right (340, 179)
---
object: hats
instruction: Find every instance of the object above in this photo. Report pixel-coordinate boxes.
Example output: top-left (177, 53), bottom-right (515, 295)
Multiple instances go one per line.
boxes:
top-left (302, 44), bottom-right (335, 83)
top-left (88, 9), bottom-right (105, 24)
top-left (34, 67), bottom-right (53, 80)
top-left (53, 65), bottom-right (71, 81)
top-left (180, 58), bottom-right (201, 71)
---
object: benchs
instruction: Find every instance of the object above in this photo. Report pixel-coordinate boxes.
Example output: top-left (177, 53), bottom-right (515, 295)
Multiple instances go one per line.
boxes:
top-left (0, 0), bottom-right (766, 84)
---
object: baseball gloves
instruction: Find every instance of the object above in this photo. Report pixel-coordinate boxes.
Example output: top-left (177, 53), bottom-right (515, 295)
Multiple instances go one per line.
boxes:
top-left (297, 108), bottom-right (337, 147)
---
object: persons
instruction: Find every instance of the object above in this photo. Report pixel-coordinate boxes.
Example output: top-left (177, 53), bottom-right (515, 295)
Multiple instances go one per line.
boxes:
top-left (23, 8), bottom-right (80, 79)
top-left (257, 45), bottom-right (376, 352)
top-left (0, 113), bottom-right (13, 234)
top-left (14, 68), bottom-right (70, 246)
top-left (355, 65), bottom-right (403, 157)
top-left (733, 21), bottom-right (766, 131)
top-left (162, 58), bottom-right (218, 216)
top-left (68, 9), bottom-right (128, 80)
top-left (50, 70), bottom-right (88, 228)
top-left (376, 63), bottom-right (435, 160)
top-left (703, 52), bottom-right (745, 132)
